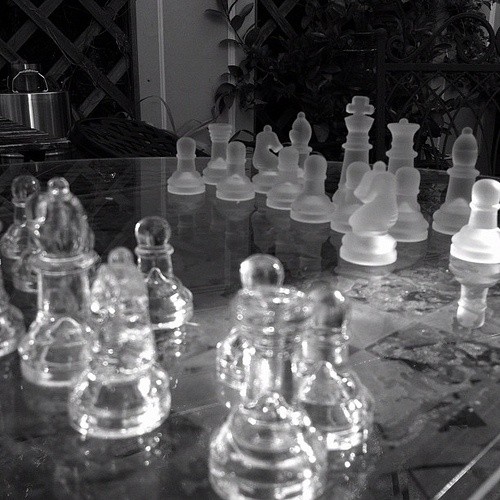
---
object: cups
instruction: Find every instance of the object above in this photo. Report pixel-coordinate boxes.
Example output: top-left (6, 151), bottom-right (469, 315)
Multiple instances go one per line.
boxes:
top-left (11, 62), bottom-right (42, 93)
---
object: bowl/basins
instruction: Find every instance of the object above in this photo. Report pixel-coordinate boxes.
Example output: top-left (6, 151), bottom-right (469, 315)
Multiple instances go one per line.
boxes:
top-left (0, 92), bottom-right (74, 147)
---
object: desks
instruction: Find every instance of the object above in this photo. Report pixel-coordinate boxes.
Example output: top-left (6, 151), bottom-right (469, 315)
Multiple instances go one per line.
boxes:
top-left (0, 158), bottom-right (500, 500)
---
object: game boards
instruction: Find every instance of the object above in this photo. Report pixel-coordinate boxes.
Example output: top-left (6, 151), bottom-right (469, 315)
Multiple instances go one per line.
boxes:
top-left (0, 156), bottom-right (499, 500)
